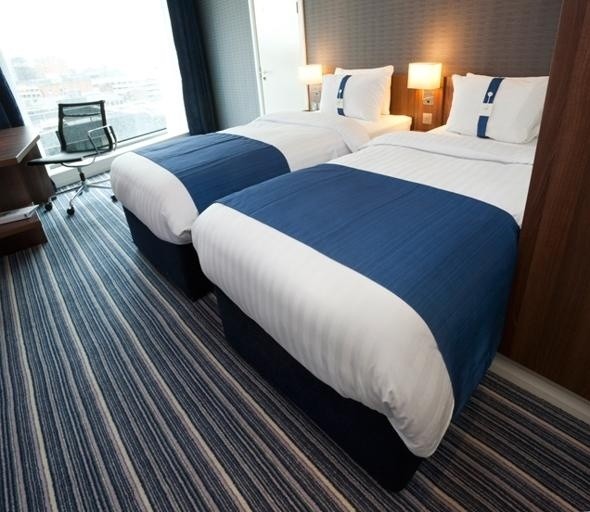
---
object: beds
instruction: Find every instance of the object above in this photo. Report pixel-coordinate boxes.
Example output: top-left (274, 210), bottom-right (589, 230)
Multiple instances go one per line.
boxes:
top-left (190, 73), bottom-right (554, 492)
top-left (111, 70), bottom-right (423, 302)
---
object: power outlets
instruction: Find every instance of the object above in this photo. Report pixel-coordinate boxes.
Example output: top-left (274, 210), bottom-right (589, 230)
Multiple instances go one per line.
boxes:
top-left (422, 112), bottom-right (432, 124)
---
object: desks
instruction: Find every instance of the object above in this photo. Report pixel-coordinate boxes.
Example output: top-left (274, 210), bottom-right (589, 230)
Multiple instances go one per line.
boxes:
top-left (0, 126), bottom-right (57, 257)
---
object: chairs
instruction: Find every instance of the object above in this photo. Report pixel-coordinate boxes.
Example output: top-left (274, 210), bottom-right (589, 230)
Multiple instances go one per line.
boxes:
top-left (26, 100), bottom-right (118, 215)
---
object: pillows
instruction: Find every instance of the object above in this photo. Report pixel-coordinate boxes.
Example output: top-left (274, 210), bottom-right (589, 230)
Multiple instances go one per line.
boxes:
top-left (334, 64), bottom-right (394, 115)
top-left (466, 72), bottom-right (549, 79)
top-left (446, 73), bottom-right (549, 144)
top-left (319, 74), bottom-right (388, 121)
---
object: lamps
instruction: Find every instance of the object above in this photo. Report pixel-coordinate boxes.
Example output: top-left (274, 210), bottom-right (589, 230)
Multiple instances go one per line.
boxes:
top-left (299, 63), bottom-right (323, 96)
top-left (407, 62), bottom-right (442, 105)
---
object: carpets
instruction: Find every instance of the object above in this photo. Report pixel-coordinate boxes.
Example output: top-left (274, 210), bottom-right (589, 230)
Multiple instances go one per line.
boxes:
top-left (0, 170), bottom-right (590, 512)
